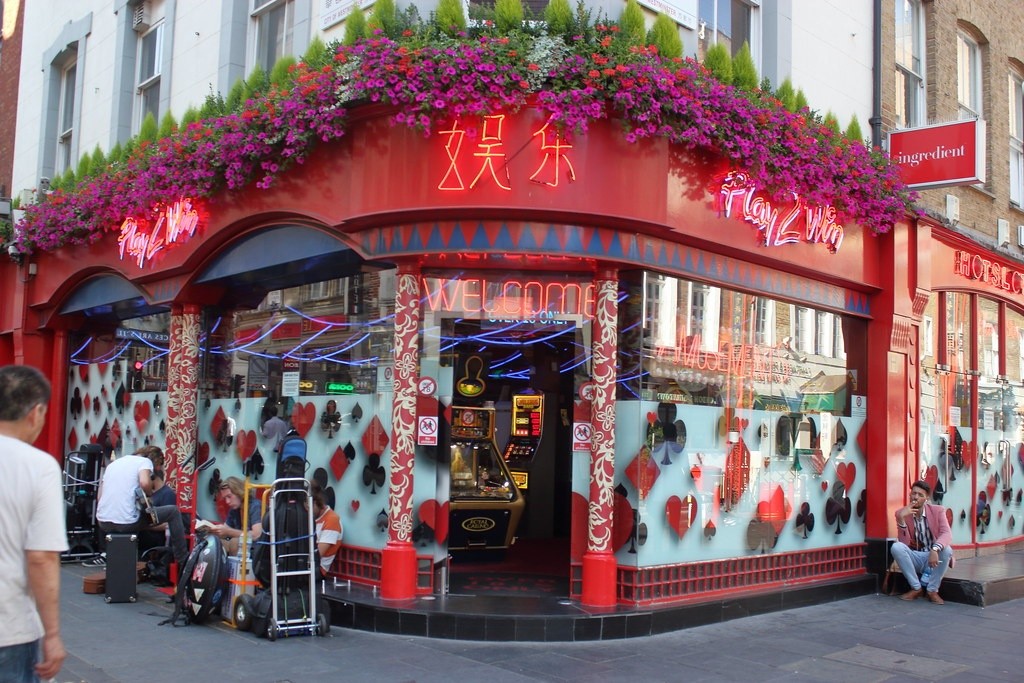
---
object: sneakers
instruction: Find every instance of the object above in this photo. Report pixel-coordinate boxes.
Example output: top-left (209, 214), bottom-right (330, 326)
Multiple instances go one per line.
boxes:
top-left (82, 554), bottom-right (107, 567)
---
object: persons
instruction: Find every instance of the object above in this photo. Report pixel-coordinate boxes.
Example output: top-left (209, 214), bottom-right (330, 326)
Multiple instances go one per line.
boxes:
top-left (0, 365), bottom-right (70, 683)
top-left (891, 480), bottom-right (955, 604)
top-left (81, 467), bottom-right (176, 574)
top-left (261, 406), bottom-right (288, 453)
top-left (95, 444), bottom-right (189, 568)
top-left (302, 479), bottom-right (344, 583)
top-left (165, 474), bottom-right (270, 603)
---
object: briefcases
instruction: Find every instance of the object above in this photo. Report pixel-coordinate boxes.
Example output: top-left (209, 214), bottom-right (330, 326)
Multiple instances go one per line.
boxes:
top-left (221, 556), bottom-right (256, 623)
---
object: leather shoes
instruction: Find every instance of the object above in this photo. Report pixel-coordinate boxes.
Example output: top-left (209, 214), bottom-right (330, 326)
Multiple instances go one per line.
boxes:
top-left (900, 588), bottom-right (924, 600)
top-left (926, 590), bottom-right (944, 605)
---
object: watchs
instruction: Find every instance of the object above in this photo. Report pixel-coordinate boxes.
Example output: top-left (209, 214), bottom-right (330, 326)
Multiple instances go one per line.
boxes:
top-left (931, 546), bottom-right (939, 551)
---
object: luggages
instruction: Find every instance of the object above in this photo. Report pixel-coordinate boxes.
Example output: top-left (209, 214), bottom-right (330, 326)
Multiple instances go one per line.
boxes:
top-left (105, 533), bottom-right (137, 603)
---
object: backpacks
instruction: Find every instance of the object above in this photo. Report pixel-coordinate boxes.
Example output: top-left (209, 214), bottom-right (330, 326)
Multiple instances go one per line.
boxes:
top-left (157, 534), bottom-right (228, 627)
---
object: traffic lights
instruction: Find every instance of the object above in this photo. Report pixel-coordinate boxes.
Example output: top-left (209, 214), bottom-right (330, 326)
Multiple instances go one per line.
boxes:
top-left (133, 360), bottom-right (143, 392)
top-left (234, 374), bottom-right (246, 398)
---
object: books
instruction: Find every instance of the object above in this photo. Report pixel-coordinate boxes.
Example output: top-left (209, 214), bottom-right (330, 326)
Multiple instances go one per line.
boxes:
top-left (194, 519), bottom-right (215, 533)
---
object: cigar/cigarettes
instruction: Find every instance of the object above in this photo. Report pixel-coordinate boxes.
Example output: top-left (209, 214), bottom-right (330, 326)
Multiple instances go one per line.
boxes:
top-left (912, 501), bottom-right (916, 504)
